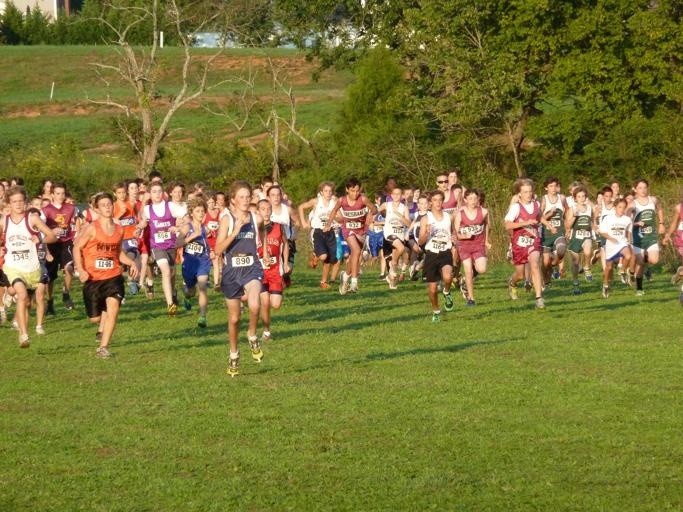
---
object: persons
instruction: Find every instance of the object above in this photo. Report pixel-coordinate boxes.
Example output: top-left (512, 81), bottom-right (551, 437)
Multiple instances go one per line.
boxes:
top-left (504, 177), bottom-right (683, 310)
top-left (0, 170), bottom-right (299, 375)
top-left (300, 170), bottom-right (492, 324)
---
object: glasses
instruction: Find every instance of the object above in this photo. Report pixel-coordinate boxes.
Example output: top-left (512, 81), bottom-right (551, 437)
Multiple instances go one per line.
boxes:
top-left (436, 180), bottom-right (448, 184)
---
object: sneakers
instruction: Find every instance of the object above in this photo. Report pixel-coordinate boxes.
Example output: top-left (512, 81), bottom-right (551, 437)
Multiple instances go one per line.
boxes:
top-left (0, 255), bottom-right (683, 377)
